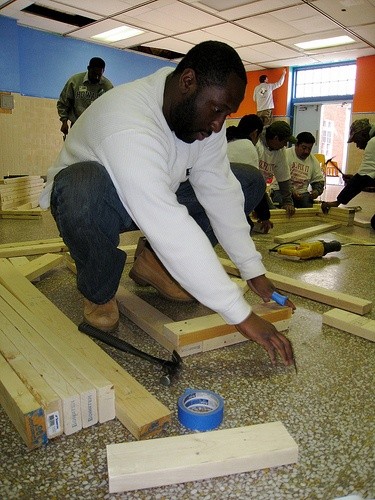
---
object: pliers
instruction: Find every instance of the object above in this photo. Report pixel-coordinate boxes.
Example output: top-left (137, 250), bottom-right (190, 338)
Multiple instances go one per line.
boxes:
top-left (271, 292), bottom-right (296, 314)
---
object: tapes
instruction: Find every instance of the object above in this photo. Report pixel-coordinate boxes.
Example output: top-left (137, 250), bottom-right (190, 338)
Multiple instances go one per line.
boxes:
top-left (177, 389), bottom-right (224, 431)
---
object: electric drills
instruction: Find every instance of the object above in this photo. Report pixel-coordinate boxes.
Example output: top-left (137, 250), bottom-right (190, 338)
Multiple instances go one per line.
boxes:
top-left (269, 239), bottom-right (354, 260)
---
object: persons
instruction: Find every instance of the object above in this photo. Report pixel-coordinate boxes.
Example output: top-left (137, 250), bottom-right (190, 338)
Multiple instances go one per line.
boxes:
top-left (253, 68), bottom-right (286, 126)
top-left (269, 132), bottom-right (325, 209)
top-left (225, 114), bottom-right (273, 234)
top-left (57, 57), bottom-right (114, 142)
top-left (40, 39), bottom-right (295, 365)
top-left (321, 118), bottom-right (375, 231)
top-left (255, 120), bottom-right (295, 217)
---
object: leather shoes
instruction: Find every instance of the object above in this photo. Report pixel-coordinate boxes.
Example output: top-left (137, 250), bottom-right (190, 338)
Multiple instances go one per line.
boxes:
top-left (133, 237), bottom-right (147, 264)
top-left (129, 247), bottom-right (196, 303)
top-left (82, 294), bottom-right (120, 332)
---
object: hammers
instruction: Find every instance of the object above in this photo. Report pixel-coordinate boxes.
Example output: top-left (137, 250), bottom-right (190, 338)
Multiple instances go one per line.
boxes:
top-left (325, 156), bottom-right (344, 175)
top-left (78, 322), bottom-right (184, 386)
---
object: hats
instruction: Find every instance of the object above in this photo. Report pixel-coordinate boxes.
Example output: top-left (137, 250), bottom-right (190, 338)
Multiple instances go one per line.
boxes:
top-left (347, 118), bottom-right (371, 143)
top-left (266, 121), bottom-right (298, 144)
